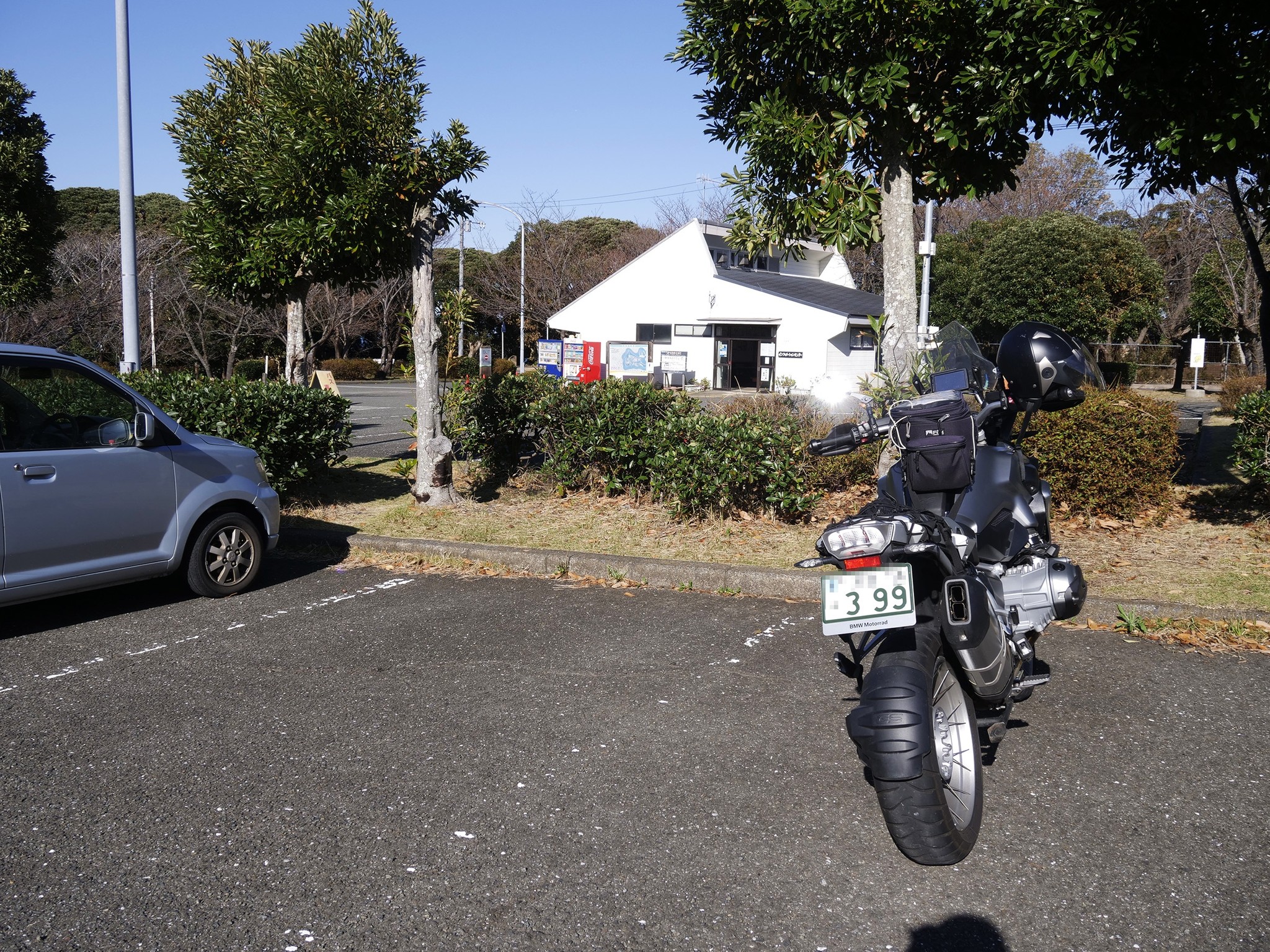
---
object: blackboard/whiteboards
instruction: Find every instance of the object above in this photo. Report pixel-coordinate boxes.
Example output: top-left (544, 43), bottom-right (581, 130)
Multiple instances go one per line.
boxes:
top-left (660, 350), bottom-right (687, 374)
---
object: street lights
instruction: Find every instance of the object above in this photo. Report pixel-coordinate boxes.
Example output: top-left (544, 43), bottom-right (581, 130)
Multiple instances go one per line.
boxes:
top-left (467, 199), bottom-right (525, 376)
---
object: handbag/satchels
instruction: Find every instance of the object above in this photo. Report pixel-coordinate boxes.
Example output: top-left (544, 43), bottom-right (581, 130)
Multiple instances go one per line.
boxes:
top-left (889, 390), bottom-right (978, 491)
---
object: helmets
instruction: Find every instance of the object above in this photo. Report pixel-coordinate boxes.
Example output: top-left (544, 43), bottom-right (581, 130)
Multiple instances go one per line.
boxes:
top-left (996, 321), bottom-right (1086, 410)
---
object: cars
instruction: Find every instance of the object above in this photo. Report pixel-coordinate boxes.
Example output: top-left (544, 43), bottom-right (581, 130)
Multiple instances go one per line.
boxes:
top-left (0, 343), bottom-right (282, 606)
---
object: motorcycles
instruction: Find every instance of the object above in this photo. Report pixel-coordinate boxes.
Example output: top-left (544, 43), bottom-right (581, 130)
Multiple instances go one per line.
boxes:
top-left (795, 318), bottom-right (1090, 866)
